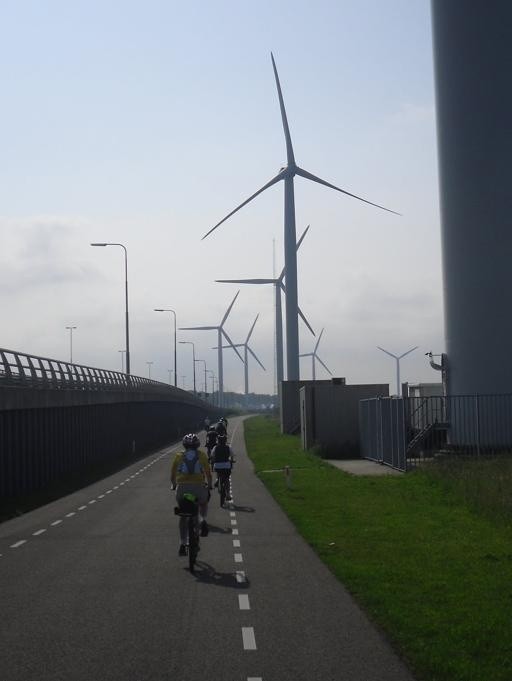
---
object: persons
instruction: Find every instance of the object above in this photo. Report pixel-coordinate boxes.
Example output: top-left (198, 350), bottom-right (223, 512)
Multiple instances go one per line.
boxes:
top-left (170, 433), bottom-right (212, 556)
top-left (200, 414), bottom-right (233, 499)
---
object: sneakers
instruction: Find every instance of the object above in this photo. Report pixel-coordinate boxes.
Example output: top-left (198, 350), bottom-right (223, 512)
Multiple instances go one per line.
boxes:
top-left (200, 520), bottom-right (208, 537)
top-left (179, 544), bottom-right (187, 557)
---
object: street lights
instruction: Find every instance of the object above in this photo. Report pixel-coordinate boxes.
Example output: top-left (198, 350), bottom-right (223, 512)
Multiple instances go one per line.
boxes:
top-left (89, 240), bottom-right (134, 376)
top-left (193, 358), bottom-right (229, 410)
top-left (179, 340), bottom-right (199, 399)
top-left (118, 348), bottom-right (125, 373)
top-left (166, 369), bottom-right (188, 390)
top-left (147, 361), bottom-right (154, 380)
top-left (66, 324), bottom-right (78, 374)
top-left (153, 308), bottom-right (178, 388)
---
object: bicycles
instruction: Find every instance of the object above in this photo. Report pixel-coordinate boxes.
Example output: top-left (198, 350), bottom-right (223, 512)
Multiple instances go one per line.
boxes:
top-left (203, 422), bottom-right (234, 506)
top-left (169, 480), bottom-right (212, 573)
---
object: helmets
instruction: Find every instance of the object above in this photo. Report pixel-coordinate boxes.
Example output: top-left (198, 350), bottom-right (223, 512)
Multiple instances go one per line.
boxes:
top-left (183, 434), bottom-right (201, 448)
